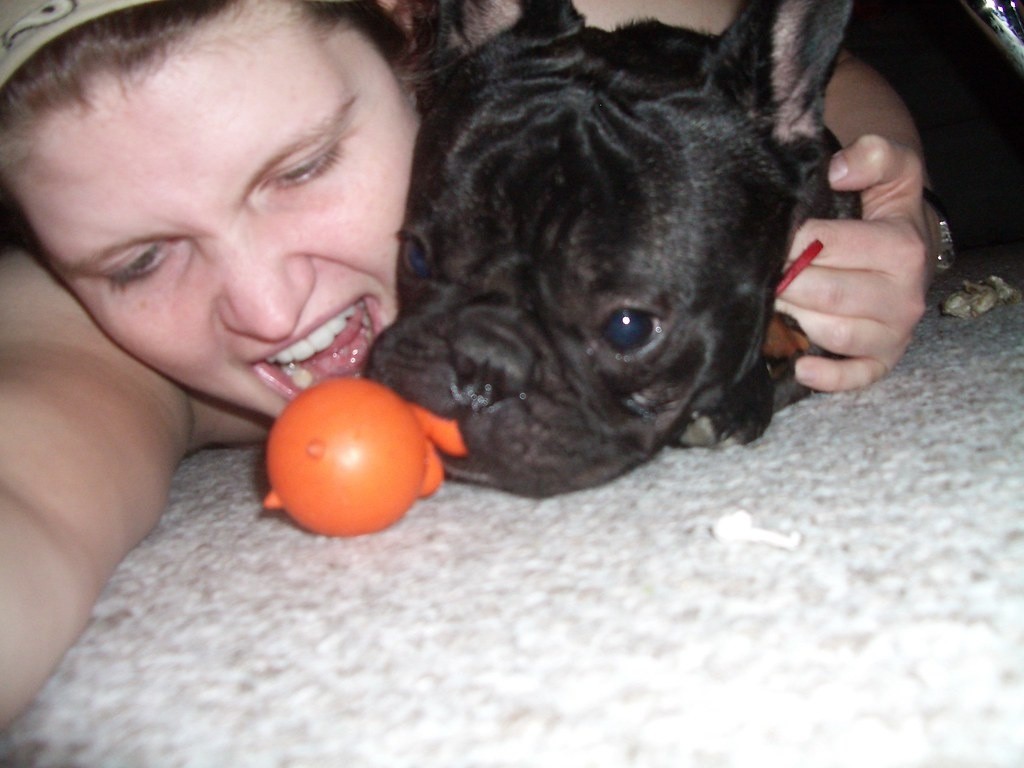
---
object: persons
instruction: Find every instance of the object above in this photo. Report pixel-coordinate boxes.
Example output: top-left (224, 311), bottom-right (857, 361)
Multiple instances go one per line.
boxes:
top-left (0, 0), bottom-right (956, 724)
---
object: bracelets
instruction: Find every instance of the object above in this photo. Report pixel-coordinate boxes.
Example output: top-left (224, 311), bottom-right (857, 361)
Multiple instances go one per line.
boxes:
top-left (922, 187), bottom-right (954, 275)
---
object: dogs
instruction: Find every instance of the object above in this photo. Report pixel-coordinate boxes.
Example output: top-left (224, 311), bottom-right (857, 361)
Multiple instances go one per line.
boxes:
top-left (362, 0), bottom-right (866, 500)
